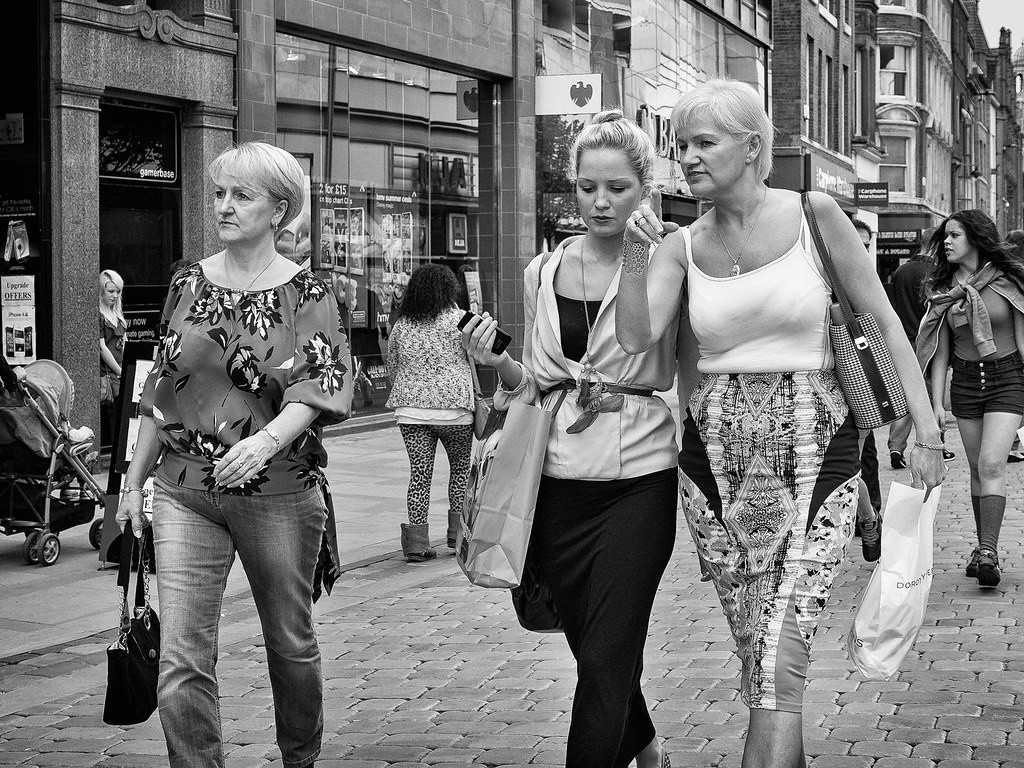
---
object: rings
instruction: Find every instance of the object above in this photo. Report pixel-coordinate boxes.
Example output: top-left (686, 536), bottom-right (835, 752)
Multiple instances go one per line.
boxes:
top-left (635, 217), bottom-right (647, 226)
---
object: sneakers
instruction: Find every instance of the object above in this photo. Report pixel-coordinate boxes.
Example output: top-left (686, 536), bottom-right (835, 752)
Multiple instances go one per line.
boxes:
top-left (858, 508), bottom-right (881, 562)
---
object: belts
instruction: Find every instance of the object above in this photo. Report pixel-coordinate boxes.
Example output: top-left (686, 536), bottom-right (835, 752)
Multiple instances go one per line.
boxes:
top-left (546, 378), bottom-right (652, 419)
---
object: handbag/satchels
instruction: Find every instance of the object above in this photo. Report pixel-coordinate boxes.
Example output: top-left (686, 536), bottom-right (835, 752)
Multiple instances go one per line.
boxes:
top-left (455, 387), bottom-right (553, 589)
top-left (102, 520), bottom-right (160, 726)
top-left (847, 467), bottom-right (950, 683)
top-left (801, 191), bottom-right (908, 430)
top-left (473, 397), bottom-right (492, 441)
top-left (100, 375), bottom-right (114, 406)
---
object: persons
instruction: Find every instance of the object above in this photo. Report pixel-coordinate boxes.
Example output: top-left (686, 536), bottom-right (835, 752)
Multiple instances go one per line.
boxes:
top-left (851, 220), bottom-right (882, 537)
top-left (917, 209), bottom-right (1024, 590)
top-left (615, 78), bottom-right (949, 768)
top-left (460, 108), bottom-right (713, 768)
top-left (169, 259), bottom-right (191, 279)
top-left (98, 270), bottom-right (127, 455)
top-left (115, 141), bottom-right (353, 768)
top-left (60, 490), bottom-right (94, 500)
top-left (0, 343), bottom-right (27, 397)
top-left (384, 262), bottom-right (476, 562)
top-left (322, 217), bottom-right (331, 234)
top-left (888, 226), bottom-right (956, 470)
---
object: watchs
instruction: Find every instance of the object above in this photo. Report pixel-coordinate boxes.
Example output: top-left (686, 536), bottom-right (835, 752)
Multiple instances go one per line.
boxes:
top-left (263, 427), bottom-right (280, 452)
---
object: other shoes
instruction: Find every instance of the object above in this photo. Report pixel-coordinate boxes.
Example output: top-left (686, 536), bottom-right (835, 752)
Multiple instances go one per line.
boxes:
top-left (975, 550), bottom-right (1002, 587)
top-left (944, 449), bottom-right (956, 461)
top-left (661, 746), bottom-right (671, 768)
top-left (1007, 452), bottom-right (1024, 462)
top-left (891, 449), bottom-right (905, 469)
top-left (966, 547), bottom-right (980, 576)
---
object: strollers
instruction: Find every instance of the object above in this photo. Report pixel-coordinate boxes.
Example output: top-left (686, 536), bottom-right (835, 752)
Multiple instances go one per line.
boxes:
top-left (0, 359), bottom-right (126, 570)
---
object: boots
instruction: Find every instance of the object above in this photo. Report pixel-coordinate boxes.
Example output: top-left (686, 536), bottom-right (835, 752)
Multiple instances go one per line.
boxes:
top-left (447, 509), bottom-right (459, 547)
top-left (401, 522), bottom-right (437, 561)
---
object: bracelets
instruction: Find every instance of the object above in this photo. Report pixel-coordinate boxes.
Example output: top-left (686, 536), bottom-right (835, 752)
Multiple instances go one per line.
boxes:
top-left (121, 487), bottom-right (148, 497)
top-left (915, 441), bottom-right (944, 449)
top-left (621, 238), bottom-right (646, 276)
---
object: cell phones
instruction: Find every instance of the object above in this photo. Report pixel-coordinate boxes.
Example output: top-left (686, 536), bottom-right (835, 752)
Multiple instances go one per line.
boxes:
top-left (457, 310), bottom-right (513, 356)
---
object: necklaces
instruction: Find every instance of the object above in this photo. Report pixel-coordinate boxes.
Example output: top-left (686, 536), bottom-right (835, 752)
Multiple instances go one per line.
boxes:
top-left (718, 186), bottom-right (766, 278)
top-left (225, 247), bottom-right (277, 295)
top-left (576, 234), bottom-right (603, 409)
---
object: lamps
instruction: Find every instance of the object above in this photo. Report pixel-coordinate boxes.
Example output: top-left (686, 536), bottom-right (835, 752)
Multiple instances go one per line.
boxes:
top-left (956, 163), bottom-right (981, 181)
